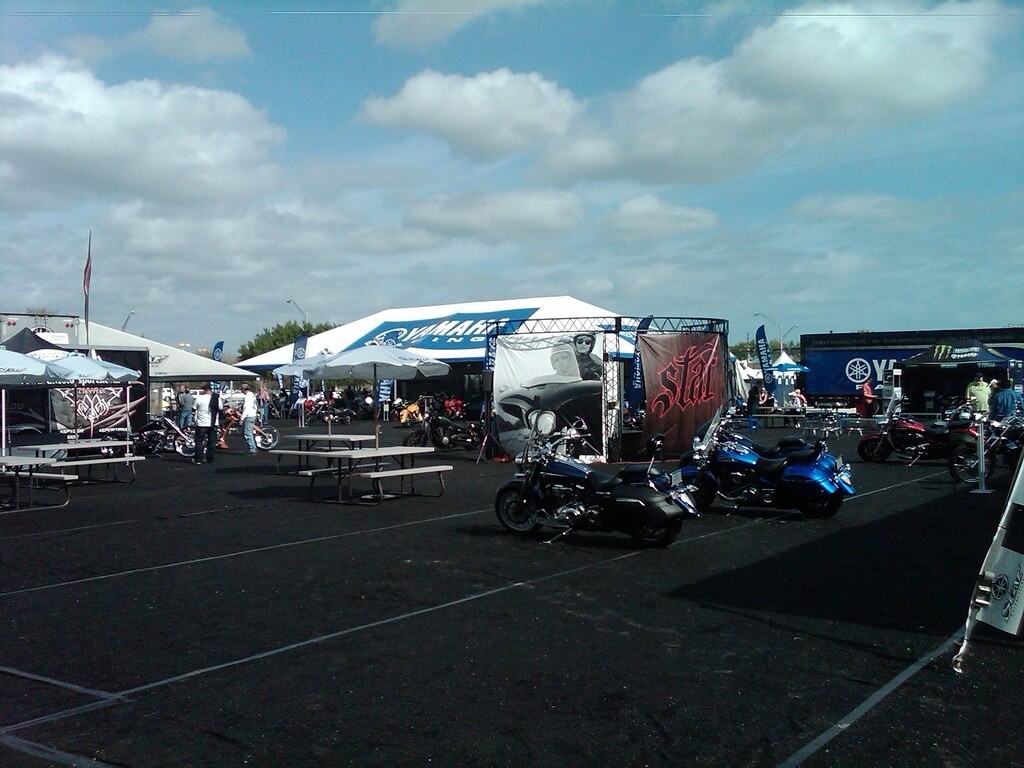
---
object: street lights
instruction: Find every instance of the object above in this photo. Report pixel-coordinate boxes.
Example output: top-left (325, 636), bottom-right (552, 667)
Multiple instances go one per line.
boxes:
top-left (754, 313), bottom-right (799, 354)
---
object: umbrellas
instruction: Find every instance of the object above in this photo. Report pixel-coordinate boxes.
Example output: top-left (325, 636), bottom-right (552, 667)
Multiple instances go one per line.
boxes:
top-left (764, 351), bottom-right (811, 388)
top-left (275, 344), bottom-right (351, 435)
top-left (306, 344), bottom-right (452, 449)
top-left (0, 348), bottom-right (140, 472)
top-left (757, 325), bottom-right (778, 392)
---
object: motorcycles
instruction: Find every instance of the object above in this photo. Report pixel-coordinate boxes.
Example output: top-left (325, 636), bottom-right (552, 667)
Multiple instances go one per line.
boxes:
top-left (100, 406), bottom-right (281, 458)
top-left (494, 408), bottom-right (700, 545)
top-left (304, 398), bottom-right (357, 426)
top-left (403, 399), bottom-right (487, 451)
top-left (671, 400), bottom-right (857, 519)
top-left (857, 393), bottom-right (1024, 483)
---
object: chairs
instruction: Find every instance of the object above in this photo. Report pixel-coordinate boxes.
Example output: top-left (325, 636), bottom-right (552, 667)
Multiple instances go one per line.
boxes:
top-left (802, 413), bottom-right (878, 438)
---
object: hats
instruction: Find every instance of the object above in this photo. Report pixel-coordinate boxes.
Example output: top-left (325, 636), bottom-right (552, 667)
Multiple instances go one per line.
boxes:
top-left (975, 372), bottom-right (983, 377)
top-left (986, 379), bottom-right (998, 387)
top-left (795, 389), bottom-right (801, 393)
top-left (240, 383), bottom-right (249, 388)
top-left (788, 392), bottom-right (797, 396)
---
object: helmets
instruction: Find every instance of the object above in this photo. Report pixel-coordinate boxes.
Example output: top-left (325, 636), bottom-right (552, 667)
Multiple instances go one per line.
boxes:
top-left (646, 435), bottom-right (665, 457)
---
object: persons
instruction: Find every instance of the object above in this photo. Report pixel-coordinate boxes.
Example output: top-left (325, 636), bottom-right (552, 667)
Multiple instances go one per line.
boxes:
top-left (255, 388), bottom-right (270, 426)
top-left (783, 389), bottom-right (806, 429)
top-left (573, 332), bottom-right (596, 355)
top-left (746, 386), bottom-right (777, 418)
top-left (175, 385), bottom-right (194, 430)
top-left (966, 372), bottom-right (1024, 420)
top-left (551, 342), bottom-right (573, 375)
top-left (240, 384), bottom-right (257, 456)
top-left (192, 384), bottom-right (223, 465)
top-left (862, 376), bottom-right (879, 418)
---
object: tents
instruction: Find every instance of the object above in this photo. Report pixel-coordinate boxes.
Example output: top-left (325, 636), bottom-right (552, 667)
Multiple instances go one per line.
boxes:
top-left (0, 277), bottom-right (668, 383)
top-left (892, 339), bottom-right (1010, 411)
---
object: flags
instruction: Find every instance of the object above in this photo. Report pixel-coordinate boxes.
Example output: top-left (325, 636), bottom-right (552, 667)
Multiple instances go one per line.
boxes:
top-left (291, 335), bottom-right (309, 404)
top-left (210, 341), bottom-right (224, 392)
top-left (83, 256), bottom-right (92, 329)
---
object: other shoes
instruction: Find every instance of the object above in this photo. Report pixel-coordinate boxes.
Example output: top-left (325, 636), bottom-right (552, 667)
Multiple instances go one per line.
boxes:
top-left (202, 460), bottom-right (215, 464)
top-left (247, 453), bottom-right (256, 456)
top-left (794, 423), bottom-right (800, 428)
top-left (192, 458), bottom-right (201, 464)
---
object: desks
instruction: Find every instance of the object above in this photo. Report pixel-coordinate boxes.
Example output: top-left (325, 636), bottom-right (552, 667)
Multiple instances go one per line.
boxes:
top-left (17, 439), bottom-right (137, 490)
top-left (757, 405), bottom-right (814, 428)
top-left (0, 456), bottom-right (70, 514)
top-left (307, 445), bottom-right (445, 505)
top-left (276, 433), bottom-right (376, 477)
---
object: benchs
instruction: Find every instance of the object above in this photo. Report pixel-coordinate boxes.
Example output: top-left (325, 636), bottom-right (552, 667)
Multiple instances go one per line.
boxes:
top-left (0, 471), bottom-right (79, 482)
top-left (299, 462), bottom-right (391, 475)
top-left (361, 465), bottom-right (453, 479)
top-left (45, 453), bottom-right (145, 467)
top-left (269, 446), bottom-right (365, 457)
top-left (901, 412), bottom-right (942, 422)
top-left (752, 414), bottom-right (805, 417)
